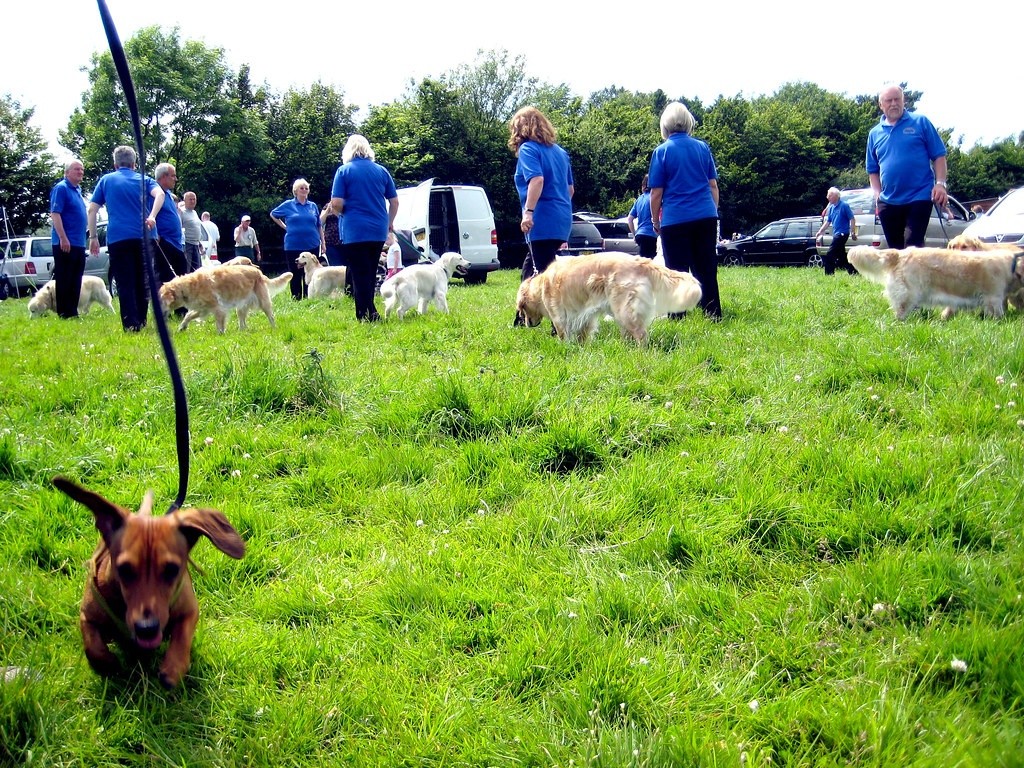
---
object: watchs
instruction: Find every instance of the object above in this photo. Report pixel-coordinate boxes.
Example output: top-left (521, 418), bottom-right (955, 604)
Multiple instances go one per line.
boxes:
top-left (89, 233), bottom-right (99, 240)
top-left (936, 181), bottom-right (948, 188)
top-left (524, 206), bottom-right (536, 213)
top-left (650, 217), bottom-right (660, 224)
top-left (257, 250), bottom-right (261, 254)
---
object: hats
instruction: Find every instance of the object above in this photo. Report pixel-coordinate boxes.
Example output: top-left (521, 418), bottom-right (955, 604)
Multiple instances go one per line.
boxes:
top-left (242, 215), bottom-right (250, 222)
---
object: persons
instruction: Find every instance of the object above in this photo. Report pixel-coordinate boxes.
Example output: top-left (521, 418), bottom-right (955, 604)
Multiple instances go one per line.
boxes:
top-left (234, 215), bottom-right (261, 265)
top-left (971, 204), bottom-right (986, 219)
top-left (863, 84), bottom-right (949, 249)
top-left (815, 187), bottom-right (861, 277)
top-left (319, 196), bottom-right (346, 265)
top-left (171, 190), bottom-right (220, 272)
top-left (330, 133), bottom-right (403, 323)
top-left (86, 146), bottom-right (165, 332)
top-left (507, 106), bottom-right (574, 327)
top-left (147, 162), bottom-right (188, 318)
top-left (626, 172), bottom-right (661, 258)
top-left (50, 159), bottom-right (88, 319)
top-left (269, 178), bottom-right (326, 301)
top-left (648, 101), bottom-right (722, 324)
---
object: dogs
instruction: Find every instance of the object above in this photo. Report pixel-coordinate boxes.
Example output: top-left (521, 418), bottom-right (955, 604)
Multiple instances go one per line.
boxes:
top-left (848, 237), bottom-right (1024, 321)
top-left (52, 475), bottom-right (246, 689)
top-left (160, 256), bottom-right (295, 333)
top-left (516, 250), bottom-right (704, 348)
top-left (295, 251), bottom-right (346, 299)
top-left (379, 252), bottom-right (471, 321)
top-left (27, 275), bottom-right (117, 320)
